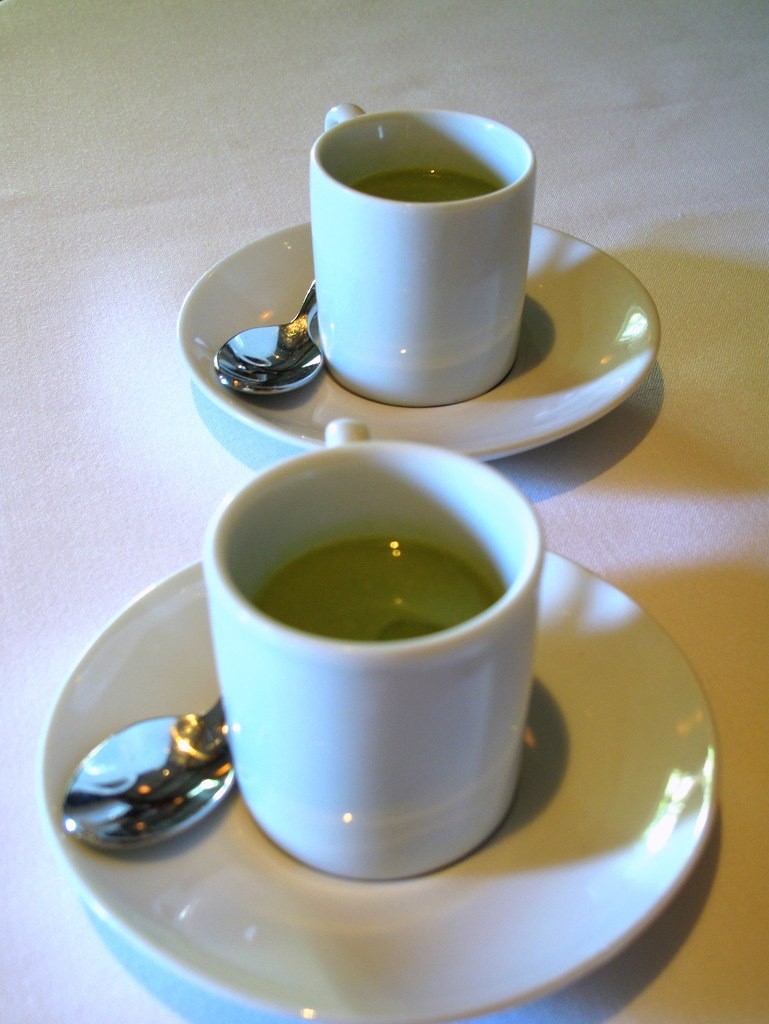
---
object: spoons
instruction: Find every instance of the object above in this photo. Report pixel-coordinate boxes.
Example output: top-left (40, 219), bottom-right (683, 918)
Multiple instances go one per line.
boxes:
top-left (214, 278), bottom-right (324, 395)
top-left (62, 695), bottom-right (236, 851)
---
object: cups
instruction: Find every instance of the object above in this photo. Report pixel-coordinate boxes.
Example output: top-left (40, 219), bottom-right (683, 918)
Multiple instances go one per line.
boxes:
top-left (308, 103), bottom-right (536, 406)
top-left (201, 419), bottom-right (545, 880)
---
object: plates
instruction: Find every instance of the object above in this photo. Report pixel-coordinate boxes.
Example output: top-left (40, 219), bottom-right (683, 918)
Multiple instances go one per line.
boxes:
top-left (176, 217), bottom-right (664, 460)
top-left (36, 548), bottom-right (720, 1024)
